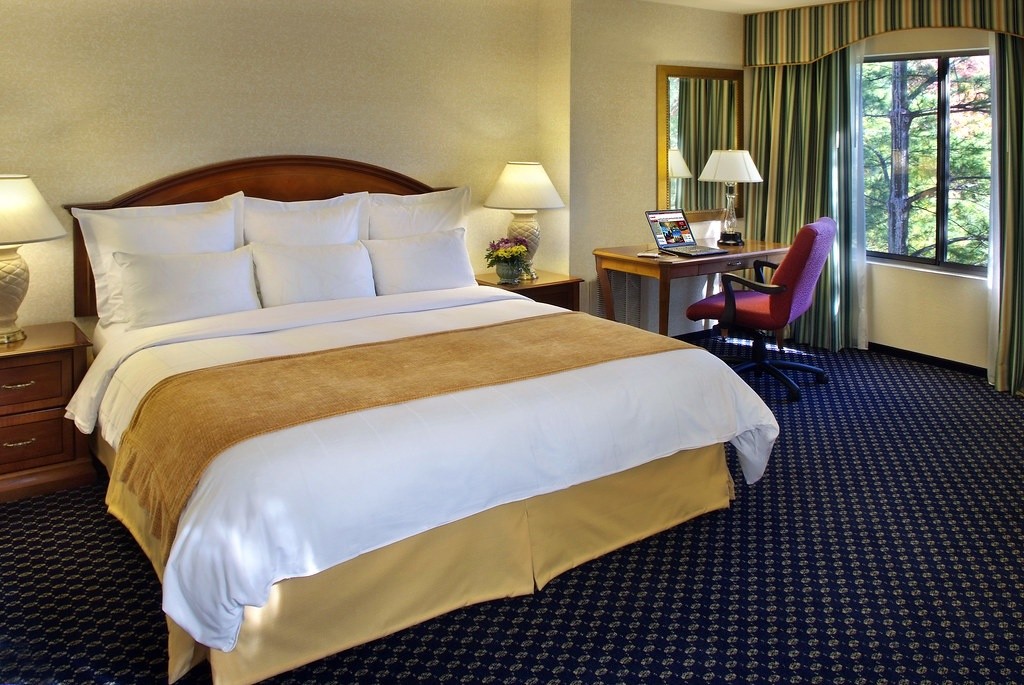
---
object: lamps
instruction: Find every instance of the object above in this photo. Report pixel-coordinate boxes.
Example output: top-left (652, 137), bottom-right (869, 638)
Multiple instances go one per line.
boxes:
top-left (0, 172), bottom-right (66, 344)
top-left (668, 148), bottom-right (693, 179)
top-left (482, 161), bottom-right (567, 280)
top-left (697, 149), bottom-right (763, 246)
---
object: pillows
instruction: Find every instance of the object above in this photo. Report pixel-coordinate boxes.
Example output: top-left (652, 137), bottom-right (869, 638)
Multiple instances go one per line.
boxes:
top-left (355, 229), bottom-right (479, 294)
top-left (112, 241), bottom-right (263, 330)
top-left (341, 181), bottom-right (472, 245)
top-left (241, 192), bottom-right (370, 249)
top-left (247, 238), bottom-right (377, 307)
top-left (71, 189), bottom-right (243, 326)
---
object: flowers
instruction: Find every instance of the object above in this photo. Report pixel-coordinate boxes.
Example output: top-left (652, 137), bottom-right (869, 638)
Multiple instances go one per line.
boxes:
top-left (486, 236), bottom-right (532, 276)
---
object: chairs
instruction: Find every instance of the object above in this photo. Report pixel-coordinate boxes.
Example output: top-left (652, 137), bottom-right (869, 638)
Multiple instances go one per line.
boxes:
top-left (685, 218), bottom-right (837, 400)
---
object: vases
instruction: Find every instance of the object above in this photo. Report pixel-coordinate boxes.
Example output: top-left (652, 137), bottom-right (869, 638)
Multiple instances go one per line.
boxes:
top-left (495, 262), bottom-right (523, 285)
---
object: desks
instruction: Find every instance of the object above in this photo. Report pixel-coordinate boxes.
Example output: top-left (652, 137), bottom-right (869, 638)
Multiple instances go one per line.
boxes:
top-left (589, 236), bottom-right (792, 341)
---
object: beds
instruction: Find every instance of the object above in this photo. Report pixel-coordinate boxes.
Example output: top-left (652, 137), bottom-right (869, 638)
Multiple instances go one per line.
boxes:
top-left (65, 155), bottom-right (778, 685)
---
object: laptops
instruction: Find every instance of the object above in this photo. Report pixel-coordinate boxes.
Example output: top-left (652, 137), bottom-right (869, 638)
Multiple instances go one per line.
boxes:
top-left (645, 209), bottom-right (729, 257)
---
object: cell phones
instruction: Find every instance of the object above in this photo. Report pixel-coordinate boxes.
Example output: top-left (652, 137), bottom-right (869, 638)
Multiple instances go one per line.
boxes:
top-left (637, 252), bottom-right (659, 258)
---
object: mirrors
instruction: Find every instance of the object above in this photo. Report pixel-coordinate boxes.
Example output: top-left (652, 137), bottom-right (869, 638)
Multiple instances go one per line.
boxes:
top-left (655, 64), bottom-right (744, 223)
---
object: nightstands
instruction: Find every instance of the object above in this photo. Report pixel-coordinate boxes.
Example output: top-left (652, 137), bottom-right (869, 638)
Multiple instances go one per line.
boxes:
top-left (476, 271), bottom-right (585, 313)
top-left (0, 321), bottom-right (98, 504)
